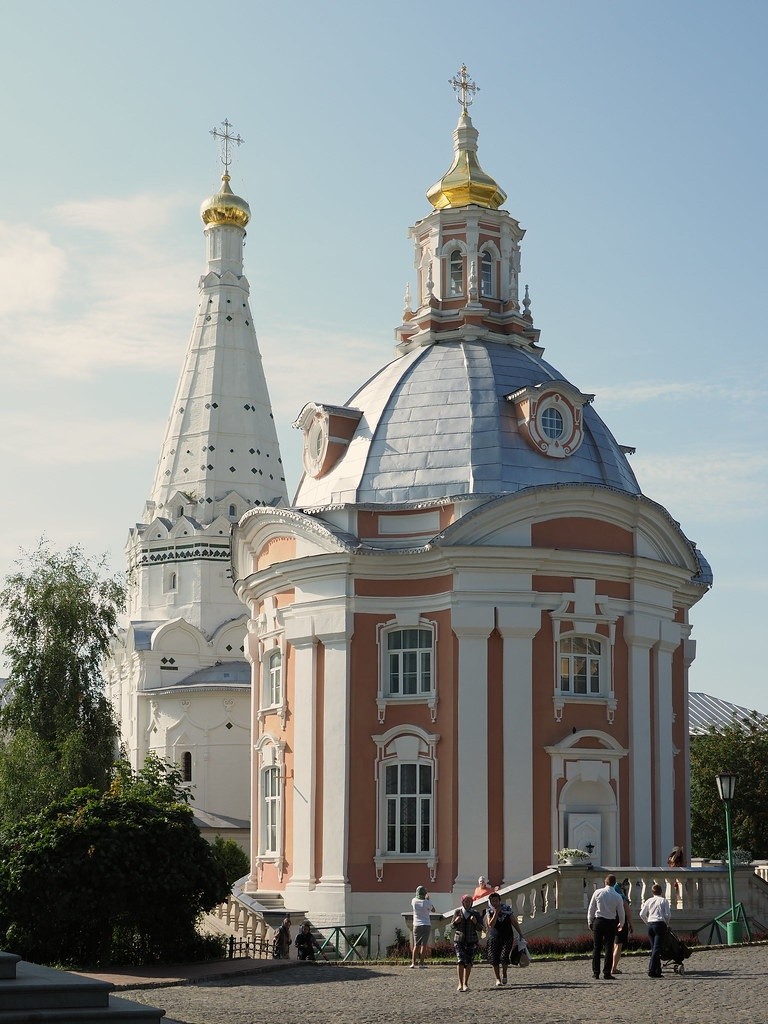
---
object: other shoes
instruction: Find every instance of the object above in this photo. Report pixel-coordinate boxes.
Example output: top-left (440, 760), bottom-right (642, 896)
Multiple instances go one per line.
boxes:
top-left (495, 979), bottom-right (501, 986)
top-left (463, 983), bottom-right (469, 991)
top-left (457, 985), bottom-right (463, 991)
top-left (611, 969), bottom-right (623, 974)
top-left (410, 965), bottom-right (414, 968)
top-left (419, 964), bottom-right (428, 968)
top-left (592, 974), bottom-right (600, 979)
top-left (604, 974), bottom-right (615, 979)
top-left (501, 974), bottom-right (507, 984)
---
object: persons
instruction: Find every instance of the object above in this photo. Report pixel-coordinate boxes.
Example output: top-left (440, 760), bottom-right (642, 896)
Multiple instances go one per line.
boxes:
top-left (586, 874), bottom-right (624, 980)
top-left (486, 892), bottom-right (523, 986)
top-left (639, 884), bottom-right (671, 978)
top-left (474, 876), bottom-right (492, 901)
top-left (275, 918), bottom-right (292, 959)
top-left (452, 894), bottom-right (483, 991)
top-left (294, 922), bottom-right (324, 960)
top-left (411, 886), bottom-right (435, 968)
top-left (668, 846), bottom-right (683, 867)
top-left (612, 883), bottom-right (633, 973)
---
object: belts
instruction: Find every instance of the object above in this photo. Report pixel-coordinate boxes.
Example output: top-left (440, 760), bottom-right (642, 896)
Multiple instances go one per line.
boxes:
top-left (648, 922), bottom-right (655, 925)
top-left (594, 917), bottom-right (602, 919)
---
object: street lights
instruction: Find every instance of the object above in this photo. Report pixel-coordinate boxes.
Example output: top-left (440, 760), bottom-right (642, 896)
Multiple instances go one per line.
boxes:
top-left (715, 770), bottom-right (744, 944)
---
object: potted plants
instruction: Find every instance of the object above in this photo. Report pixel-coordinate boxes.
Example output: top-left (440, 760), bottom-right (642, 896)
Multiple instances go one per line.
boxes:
top-left (716, 846), bottom-right (754, 867)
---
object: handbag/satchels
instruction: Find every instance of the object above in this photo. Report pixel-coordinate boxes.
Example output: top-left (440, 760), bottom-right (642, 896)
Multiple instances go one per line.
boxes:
top-left (454, 931), bottom-right (465, 943)
top-left (519, 947), bottom-right (531, 967)
top-left (509, 945), bottom-right (520, 965)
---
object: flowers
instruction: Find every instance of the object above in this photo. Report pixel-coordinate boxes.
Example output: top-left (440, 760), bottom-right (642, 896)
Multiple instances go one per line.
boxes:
top-left (554, 847), bottom-right (590, 862)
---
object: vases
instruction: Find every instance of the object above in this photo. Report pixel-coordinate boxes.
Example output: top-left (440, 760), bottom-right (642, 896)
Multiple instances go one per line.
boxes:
top-left (563, 856), bottom-right (581, 863)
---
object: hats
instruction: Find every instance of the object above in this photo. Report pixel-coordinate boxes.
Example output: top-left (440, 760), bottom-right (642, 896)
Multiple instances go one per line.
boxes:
top-left (478, 876), bottom-right (487, 886)
top-left (416, 886), bottom-right (427, 899)
